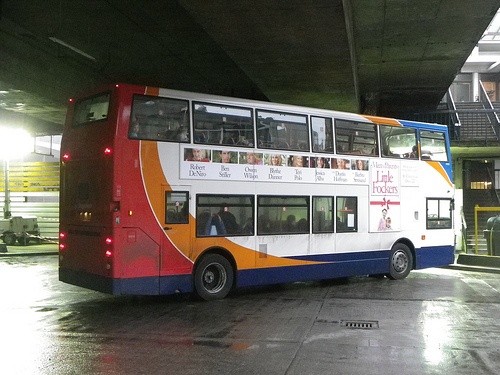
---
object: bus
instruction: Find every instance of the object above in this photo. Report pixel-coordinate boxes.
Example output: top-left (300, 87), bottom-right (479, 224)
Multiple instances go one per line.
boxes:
top-left (57, 82), bottom-right (464, 302)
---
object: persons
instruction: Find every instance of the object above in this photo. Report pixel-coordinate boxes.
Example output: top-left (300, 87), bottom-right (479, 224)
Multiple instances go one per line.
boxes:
top-left (131, 110), bottom-right (433, 159)
top-left (167, 201), bottom-right (347, 234)
top-left (376, 209), bottom-right (392, 231)
top-left (184, 148), bottom-right (366, 170)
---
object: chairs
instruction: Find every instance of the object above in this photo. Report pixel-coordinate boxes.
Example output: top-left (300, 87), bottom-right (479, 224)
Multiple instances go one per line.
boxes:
top-left (197, 209), bottom-right (213, 235)
top-left (213, 213), bottom-right (227, 234)
